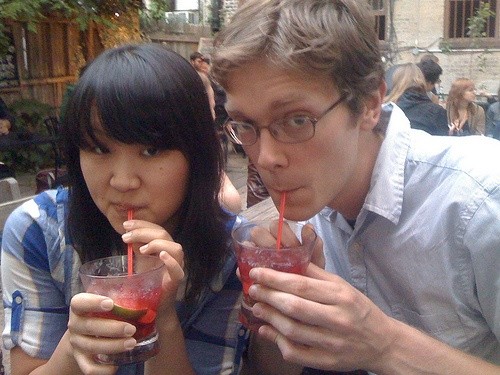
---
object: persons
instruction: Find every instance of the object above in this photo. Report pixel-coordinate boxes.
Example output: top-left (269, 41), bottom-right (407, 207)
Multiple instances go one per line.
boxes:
top-left (189, 51), bottom-right (500, 209)
top-left (0, 43), bottom-right (251, 375)
top-left (209, 0), bottom-right (500, 375)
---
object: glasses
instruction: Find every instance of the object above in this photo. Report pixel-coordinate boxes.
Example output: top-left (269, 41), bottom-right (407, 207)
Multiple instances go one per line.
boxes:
top-left (221, 93), bottom-right (346, 147)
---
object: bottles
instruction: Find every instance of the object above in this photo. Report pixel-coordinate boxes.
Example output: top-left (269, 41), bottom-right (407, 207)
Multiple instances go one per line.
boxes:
top-left (438, 85), bottom-right (446, 107)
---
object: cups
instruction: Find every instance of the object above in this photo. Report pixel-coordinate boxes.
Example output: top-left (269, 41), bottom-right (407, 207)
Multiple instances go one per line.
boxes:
top-left (232, 220), bottom-right (317, 333)
top-left (79, 254), bottom-right (166, 364)
